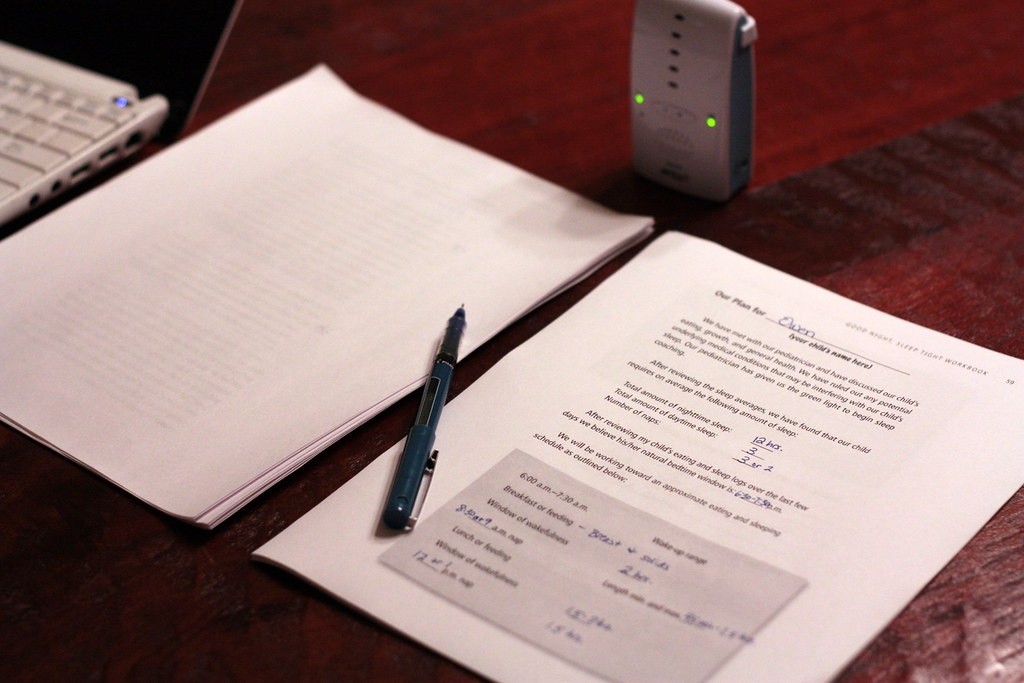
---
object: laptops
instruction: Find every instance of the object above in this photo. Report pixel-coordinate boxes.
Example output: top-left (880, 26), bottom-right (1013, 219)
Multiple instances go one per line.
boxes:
top-left (0, 0), bottom-right (238, 225)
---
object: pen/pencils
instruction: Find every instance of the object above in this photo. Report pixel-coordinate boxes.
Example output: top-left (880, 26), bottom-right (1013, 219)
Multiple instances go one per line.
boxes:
top-left (382, 302), bottom-right (466, 529)
